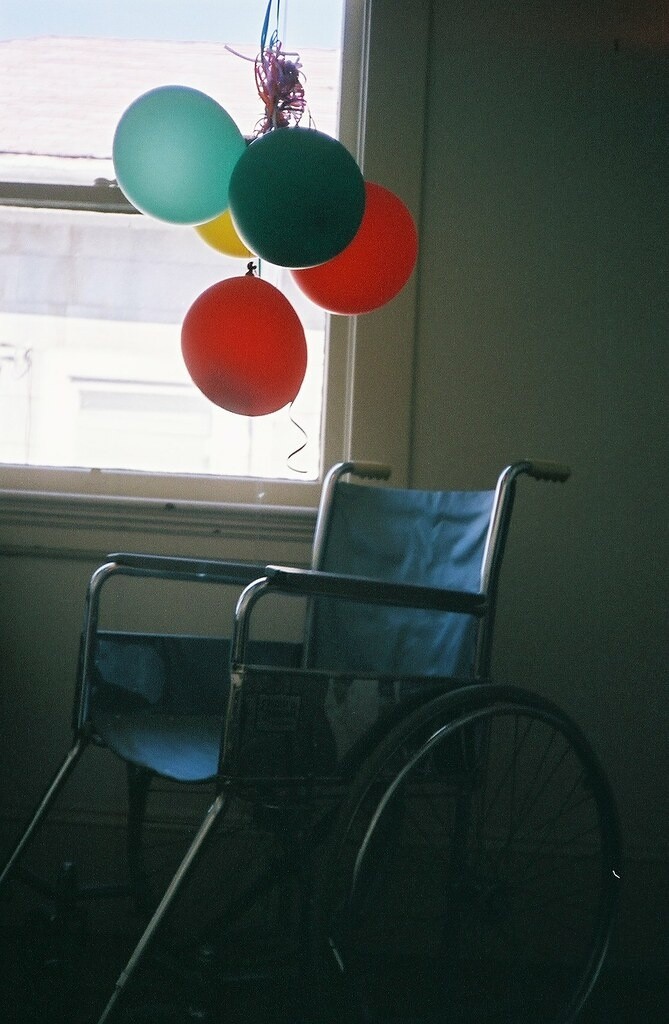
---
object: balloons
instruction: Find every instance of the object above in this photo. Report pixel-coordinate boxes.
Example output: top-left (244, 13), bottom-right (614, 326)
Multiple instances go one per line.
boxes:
top-left (192, 210), bottom-right (260, 260)
top-left (228, 127), bottom-right (367, 269)
top-left (289, 182), bottom-right (418, 316)
top-left (180, 262), bottom-right (307, 417)
top-left (113, 84), bottom-right (246, 228)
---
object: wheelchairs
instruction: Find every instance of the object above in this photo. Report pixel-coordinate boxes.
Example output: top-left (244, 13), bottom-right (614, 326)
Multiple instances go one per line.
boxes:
top-left (0, 459), bottom-right (623, 1024)
